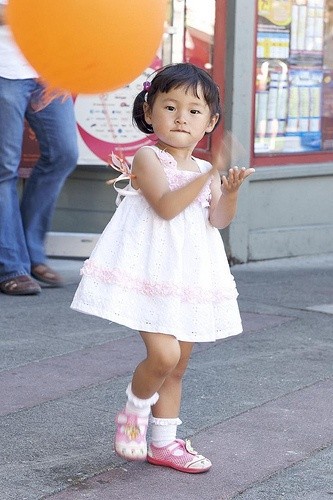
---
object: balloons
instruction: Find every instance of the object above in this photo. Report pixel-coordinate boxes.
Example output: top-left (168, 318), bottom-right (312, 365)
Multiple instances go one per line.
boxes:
top-left (8, 0), bottom-right (170, 96)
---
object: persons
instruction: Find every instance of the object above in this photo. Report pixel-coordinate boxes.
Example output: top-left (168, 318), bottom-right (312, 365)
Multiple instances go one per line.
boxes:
top-left (68, 62), bottom-right (256, 475)
top-left (0, 0), bottom-right (80, 296)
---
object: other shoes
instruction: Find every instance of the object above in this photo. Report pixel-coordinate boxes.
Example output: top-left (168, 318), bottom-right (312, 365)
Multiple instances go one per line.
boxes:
top-left (149, 436), bottom-right (212, 477)
top-left (116, 405), bottom-right (151, 463)
top-left (32, 265), bottom-right (64, 284)
top-left (2, 275), bottom-right (41, 294)
